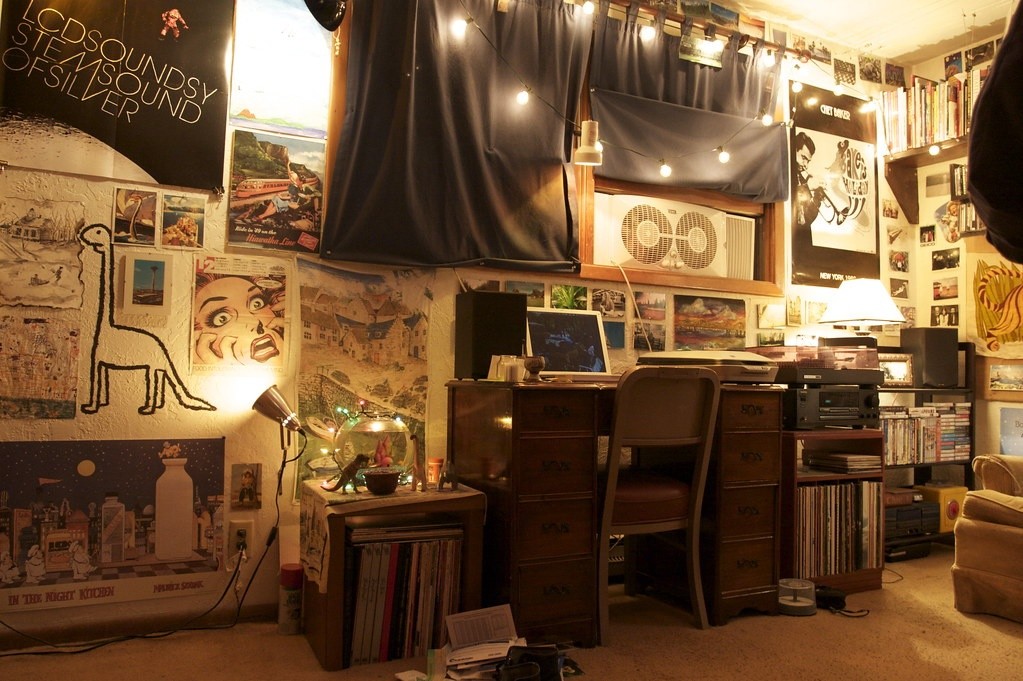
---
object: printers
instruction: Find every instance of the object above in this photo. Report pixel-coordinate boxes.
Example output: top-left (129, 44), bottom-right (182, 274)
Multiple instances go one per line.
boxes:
top-left (636, 350), bottom-right (779, 386)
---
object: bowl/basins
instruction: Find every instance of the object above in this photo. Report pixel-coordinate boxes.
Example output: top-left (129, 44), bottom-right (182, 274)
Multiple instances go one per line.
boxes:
top-left (363, 470), bottom-right (401, 495)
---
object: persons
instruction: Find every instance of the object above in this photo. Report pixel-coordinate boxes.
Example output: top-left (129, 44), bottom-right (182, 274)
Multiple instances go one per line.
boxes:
top-left (796, 132), bottom-right (829, 245)
top-left (931, 306), bottom-right (958, 326)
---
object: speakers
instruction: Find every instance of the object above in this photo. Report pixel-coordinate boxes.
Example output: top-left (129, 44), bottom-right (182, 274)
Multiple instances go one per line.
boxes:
top-left (454, 290), bottom-right (528, 380)
top-left (900, 328), bottom-right (959, 389)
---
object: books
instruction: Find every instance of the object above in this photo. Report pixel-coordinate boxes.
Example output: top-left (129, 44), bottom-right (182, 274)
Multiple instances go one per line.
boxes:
top-left (797, 481), bottom-right (882, 579)
top-left (879, 402), bottom-right (972, 466)
top-left (346, 512), bottom-right (466, 664)
top-left (812, 453), bottom-right (881, 473)
top-left (883, 62), bottom-right (986, 155)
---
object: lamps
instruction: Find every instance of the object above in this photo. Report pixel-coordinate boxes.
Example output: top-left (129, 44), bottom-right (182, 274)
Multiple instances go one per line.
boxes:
top-left (572, 117), bottom-right (604, 168)
top-left (251, 383), bottom-right (306, 451)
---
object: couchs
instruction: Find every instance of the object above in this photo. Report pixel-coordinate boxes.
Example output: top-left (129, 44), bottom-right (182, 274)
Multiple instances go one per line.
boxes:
top-left (950, 455), bottom-right (1023, 625)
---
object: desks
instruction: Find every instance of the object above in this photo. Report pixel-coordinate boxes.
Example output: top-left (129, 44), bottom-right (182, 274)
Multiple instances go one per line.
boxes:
top-left (296, 478), bottom-right (486, 672)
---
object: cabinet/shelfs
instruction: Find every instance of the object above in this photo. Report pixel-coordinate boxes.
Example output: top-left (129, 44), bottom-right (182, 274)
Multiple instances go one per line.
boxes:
top-left (871, 340), bottom-right (978, 549)
top-left (781, 427), bottom-right (886, 602)
top-left (443, 376), bottom-right (787, 651)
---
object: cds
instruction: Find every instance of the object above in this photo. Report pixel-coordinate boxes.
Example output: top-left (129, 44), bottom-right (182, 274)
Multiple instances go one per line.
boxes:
top-left (779, 595), bottom-right (814, 607)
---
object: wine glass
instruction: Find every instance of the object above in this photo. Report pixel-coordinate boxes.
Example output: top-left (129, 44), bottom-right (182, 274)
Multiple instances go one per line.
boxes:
top-left (524, 357), bottom-right (546, 381)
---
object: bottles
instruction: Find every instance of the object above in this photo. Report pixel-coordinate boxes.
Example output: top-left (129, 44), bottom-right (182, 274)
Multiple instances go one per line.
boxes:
top-left (279, 564), bottom-right (305, 634)
top-left (497, 355), bottom-right (518, 382)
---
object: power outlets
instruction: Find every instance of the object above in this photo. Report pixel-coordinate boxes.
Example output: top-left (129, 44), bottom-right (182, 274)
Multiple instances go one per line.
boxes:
top-left (228, 520), bottom-right (253, 562)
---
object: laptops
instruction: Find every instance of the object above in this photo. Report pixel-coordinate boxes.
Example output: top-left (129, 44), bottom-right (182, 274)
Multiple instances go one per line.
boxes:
top-left (525, 307), bottom-right (621, 383)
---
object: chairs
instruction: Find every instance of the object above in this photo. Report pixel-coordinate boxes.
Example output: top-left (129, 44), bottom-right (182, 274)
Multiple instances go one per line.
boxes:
top-left (595, 365), bottom-right (720, 646)
